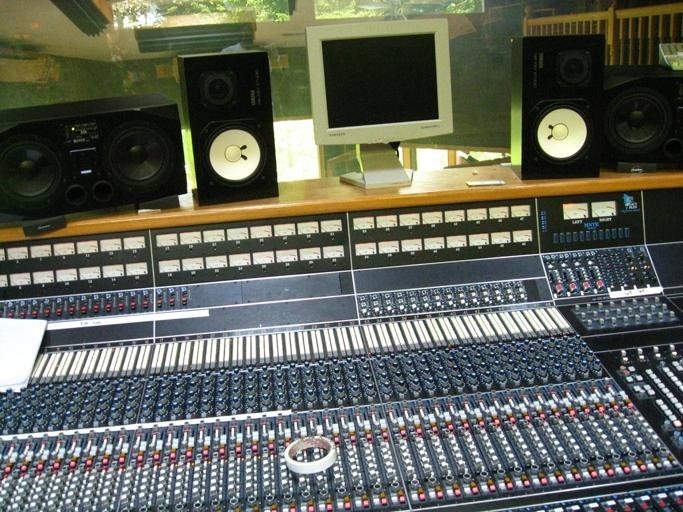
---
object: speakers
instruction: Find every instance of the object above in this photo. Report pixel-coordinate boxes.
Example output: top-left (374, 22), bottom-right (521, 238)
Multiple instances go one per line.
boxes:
top-left (601, 64), bottom-right (683, 174)
top-left (177, 47), bottom-right (279, 207)
top-left (0, 91), bottom-right (188, 239)
top-left (509, 33), bottom-right (606, 181)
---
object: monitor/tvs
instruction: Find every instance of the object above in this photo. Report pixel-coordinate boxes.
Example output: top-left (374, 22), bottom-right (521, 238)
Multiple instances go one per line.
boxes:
top-left (303, 17), bottom-right (454, 188)
